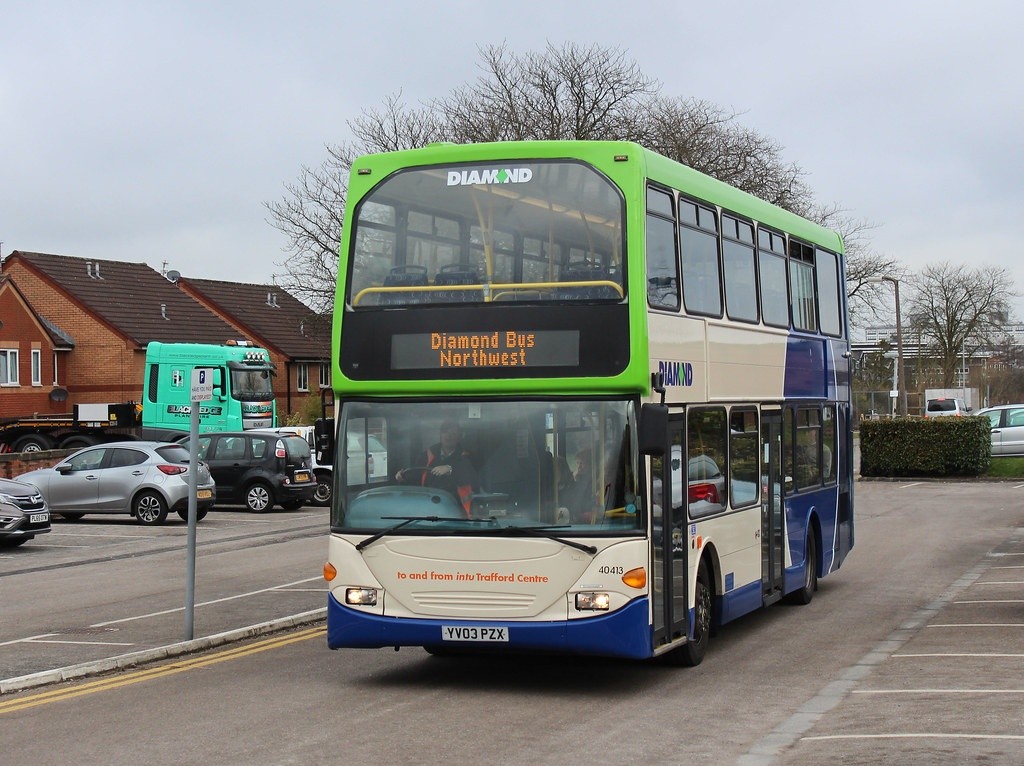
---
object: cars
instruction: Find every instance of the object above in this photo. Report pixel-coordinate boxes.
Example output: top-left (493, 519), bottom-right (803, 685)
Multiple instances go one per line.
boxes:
top-left (687, 456), bottom-right (725, 516)
top-left (761, 474), bottom-right (792, 535)
top-left (176, 431), bottom-right (319, 514)
top-left (225, 425), bottom-right (316, 450)
top-left (0, 477), bottom-right (51, 549)
top-left (971, 404), bottom-right (1024, 454)
top-left (11, 441), bottom-right (217, 525)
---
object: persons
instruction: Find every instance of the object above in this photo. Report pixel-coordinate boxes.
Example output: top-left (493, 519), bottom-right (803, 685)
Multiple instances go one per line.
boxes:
top-left (805, 423), bottom-right (832, 483)
top-left (395, 420), bottom-right (477, 519)
top-left (561, 448), bottom-right (595, 507)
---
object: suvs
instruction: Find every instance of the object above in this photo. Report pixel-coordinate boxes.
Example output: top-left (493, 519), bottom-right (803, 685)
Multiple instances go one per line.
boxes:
top-left (311, 432), bottom-right (389, 507)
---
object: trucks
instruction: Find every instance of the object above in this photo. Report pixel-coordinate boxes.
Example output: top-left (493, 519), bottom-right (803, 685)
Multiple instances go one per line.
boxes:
top-left (924, 387), bottom-right (980, 415)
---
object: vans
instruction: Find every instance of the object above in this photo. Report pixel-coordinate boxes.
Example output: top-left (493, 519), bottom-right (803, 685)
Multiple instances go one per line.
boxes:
top-left (925, 398), bottom-right (973, 417)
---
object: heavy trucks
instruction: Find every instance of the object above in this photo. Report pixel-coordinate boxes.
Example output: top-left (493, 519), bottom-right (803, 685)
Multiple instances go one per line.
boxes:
top-left (0, 339), bottom-right (279, 453)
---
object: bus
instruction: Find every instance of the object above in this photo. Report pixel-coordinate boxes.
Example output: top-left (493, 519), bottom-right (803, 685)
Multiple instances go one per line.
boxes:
top-left (313, 141), bottom-right (855, 667)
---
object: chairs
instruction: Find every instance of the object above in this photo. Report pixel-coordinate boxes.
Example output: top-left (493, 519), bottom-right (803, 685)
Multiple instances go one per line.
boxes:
top-left (254, 443), bottom-right (265, 456)
top-left (431, 263), bottom-right (484, 303)
top-left (231, 439), bottom-right (245, 457)
top-left (594, 265), bottom-right (623, 300)
top-left (555, 260), bottom-right (612, 299)
top-left (377, 265), bottom-right (430, 305)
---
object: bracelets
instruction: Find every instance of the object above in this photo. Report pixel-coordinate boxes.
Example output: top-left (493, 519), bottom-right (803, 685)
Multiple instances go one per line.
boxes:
top-left (448, 465), bottom-right (452, 474)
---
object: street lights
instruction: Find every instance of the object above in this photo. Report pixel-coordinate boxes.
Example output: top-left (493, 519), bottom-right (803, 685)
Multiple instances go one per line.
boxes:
top-left (882, 353), bottom-right (899, 420)
top-left (864, 276), bottom-right (908, 418)
top-left (959, 331), bottom-right (965, 402)
